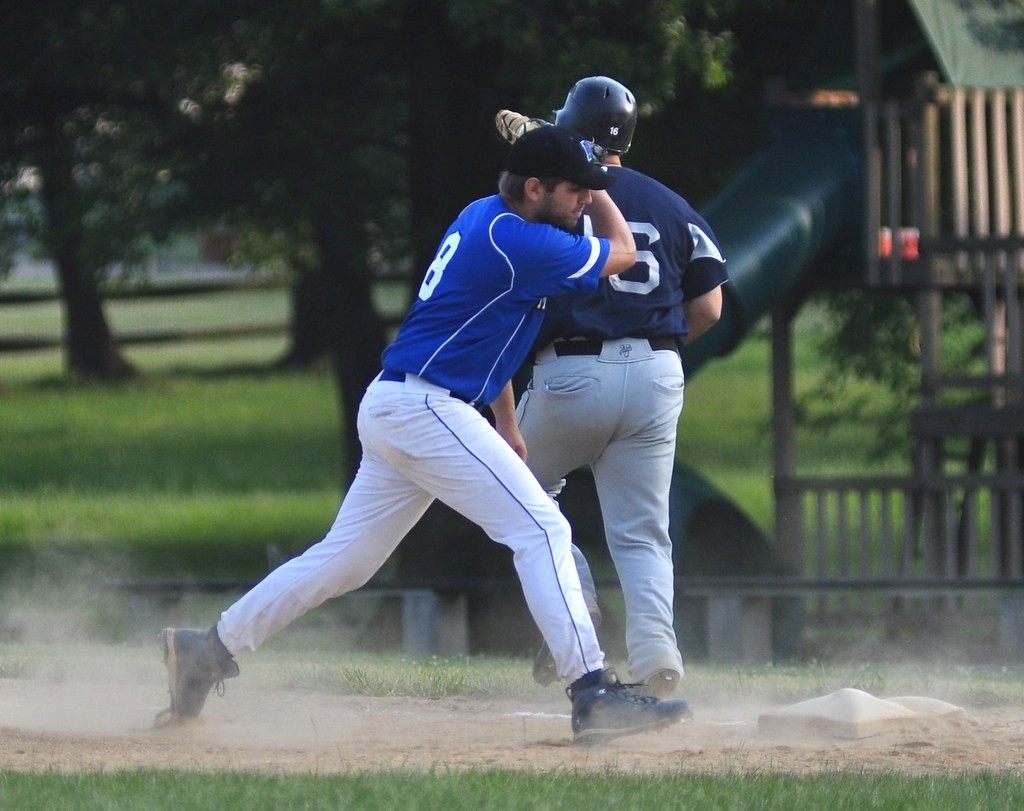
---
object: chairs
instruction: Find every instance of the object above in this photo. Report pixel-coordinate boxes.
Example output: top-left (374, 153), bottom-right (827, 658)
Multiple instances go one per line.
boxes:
top-left (888, 232), bottom-right (1023, 623)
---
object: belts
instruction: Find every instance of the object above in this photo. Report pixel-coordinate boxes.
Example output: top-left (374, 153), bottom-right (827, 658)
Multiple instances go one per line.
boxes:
top-left (554, 333), bottom-right (680, 357)
top-left (379, 371), bottom-right (472, 405)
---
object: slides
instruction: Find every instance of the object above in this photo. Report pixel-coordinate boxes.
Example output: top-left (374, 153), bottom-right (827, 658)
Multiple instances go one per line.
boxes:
top-left (390, 114), bottom-right (869, 585)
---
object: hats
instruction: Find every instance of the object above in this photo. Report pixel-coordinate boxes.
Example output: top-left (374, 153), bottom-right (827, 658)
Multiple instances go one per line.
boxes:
top-left (504, 126), bottom-right (617, 190)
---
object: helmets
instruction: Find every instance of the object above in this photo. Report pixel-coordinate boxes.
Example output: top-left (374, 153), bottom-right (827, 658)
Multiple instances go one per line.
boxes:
top-left (555, 76), bottom-right (636, 155)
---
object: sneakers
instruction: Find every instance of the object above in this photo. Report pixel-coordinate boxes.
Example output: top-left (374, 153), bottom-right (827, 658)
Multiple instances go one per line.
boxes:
top-left (533, 607), bottom-right (601, 685)
top-left (568, 669), bottom-right (690, 742)
top-left (162, 624), bottom-right (240, 721)
top-left (633, 668), bottom-right (681, 699)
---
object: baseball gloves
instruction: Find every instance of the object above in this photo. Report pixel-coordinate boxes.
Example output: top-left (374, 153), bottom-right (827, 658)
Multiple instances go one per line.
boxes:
top-left (491, 105), bottom-right (541, 142)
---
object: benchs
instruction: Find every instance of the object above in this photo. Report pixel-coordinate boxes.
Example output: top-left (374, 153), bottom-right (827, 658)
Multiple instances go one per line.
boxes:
top-left (87, 577), bottom-right (1024, 674)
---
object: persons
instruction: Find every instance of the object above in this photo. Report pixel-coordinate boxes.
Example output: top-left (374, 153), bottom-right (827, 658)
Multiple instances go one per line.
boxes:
top-left (516, 74), bottom-right (728, 699)
top-left (155, 110), bottom-right (690, 742)
